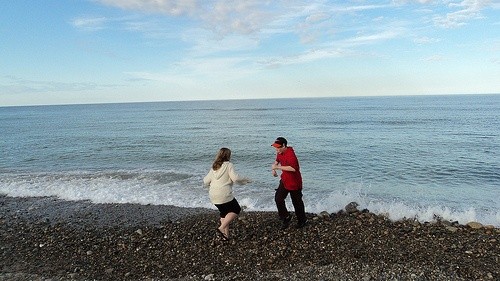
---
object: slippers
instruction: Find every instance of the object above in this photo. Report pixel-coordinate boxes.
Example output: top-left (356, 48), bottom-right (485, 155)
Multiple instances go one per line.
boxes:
top-left (217, 228), bottom-right (228, 241)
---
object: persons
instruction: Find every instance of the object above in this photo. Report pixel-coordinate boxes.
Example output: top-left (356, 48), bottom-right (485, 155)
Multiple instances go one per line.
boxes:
top-left (271, 137), bottom-right (309, 230)
top-left (203, 148), bottom-right (244, 241)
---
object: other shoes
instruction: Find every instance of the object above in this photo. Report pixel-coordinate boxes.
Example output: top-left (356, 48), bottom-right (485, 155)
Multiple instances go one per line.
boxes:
top-left (295, 219), bottom-right (309, 229)
top-left (282, 213), bottom-right (292, 229)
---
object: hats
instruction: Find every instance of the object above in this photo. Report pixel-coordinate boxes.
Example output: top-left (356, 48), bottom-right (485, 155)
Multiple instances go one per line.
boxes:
top-left (271, 137), bottom-right (287, 148)
